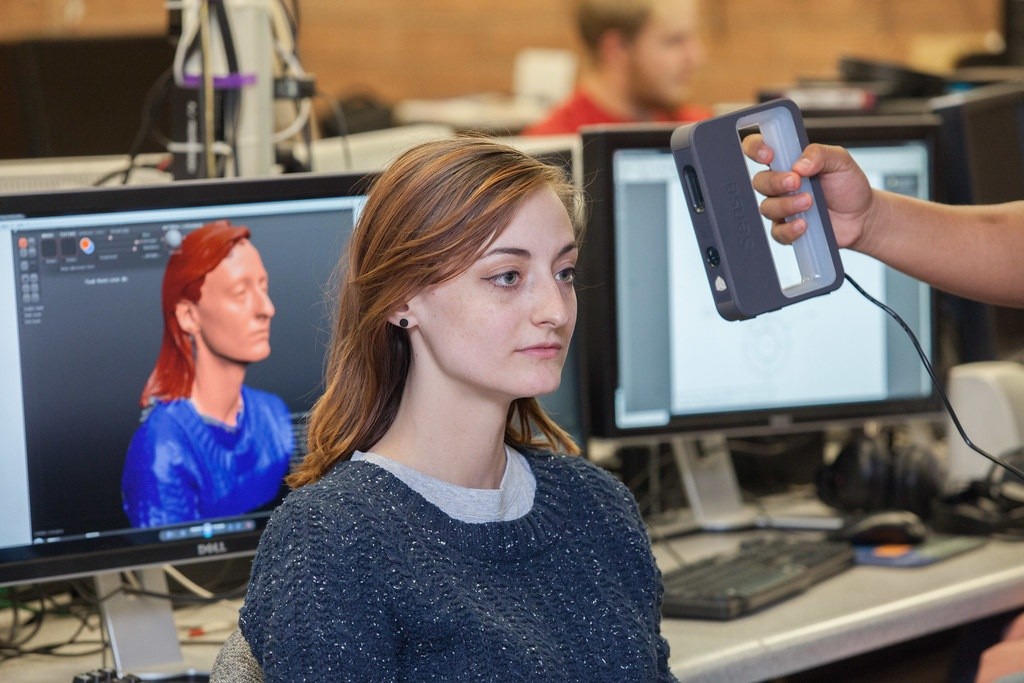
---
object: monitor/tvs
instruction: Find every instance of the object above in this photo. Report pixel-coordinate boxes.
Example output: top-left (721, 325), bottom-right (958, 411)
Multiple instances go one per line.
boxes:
top-left (512, 86), bottom-right (1024, 541)
top-left (0, 170), bottom-right (382, 683)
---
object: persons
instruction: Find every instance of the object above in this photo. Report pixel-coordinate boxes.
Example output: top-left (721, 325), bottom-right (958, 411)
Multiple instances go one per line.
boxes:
top-left (517, 0), bottom-right (718, 137)
top-left (739, 129), bottom-right (1023, 309)
top-left (203, 136), bottom-right (680, 683)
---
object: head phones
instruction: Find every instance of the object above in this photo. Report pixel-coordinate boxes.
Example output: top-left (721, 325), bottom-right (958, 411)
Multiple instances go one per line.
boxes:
top-left (896, 446), bottom-right (1024, 538)
top-left (816, 424), bottom-right (942, 514)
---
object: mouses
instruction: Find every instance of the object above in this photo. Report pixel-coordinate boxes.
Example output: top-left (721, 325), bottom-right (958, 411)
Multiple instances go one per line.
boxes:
top-left (832, 510), bottom-right (928, 545)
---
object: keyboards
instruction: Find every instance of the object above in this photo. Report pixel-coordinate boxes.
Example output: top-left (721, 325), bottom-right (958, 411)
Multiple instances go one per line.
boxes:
top-left (660, 539), bottom-right (855, 623)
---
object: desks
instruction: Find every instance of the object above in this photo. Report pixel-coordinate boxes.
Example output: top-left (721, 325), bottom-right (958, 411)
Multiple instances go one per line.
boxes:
top-left (0, 99), bottom-right (1024, 683)
